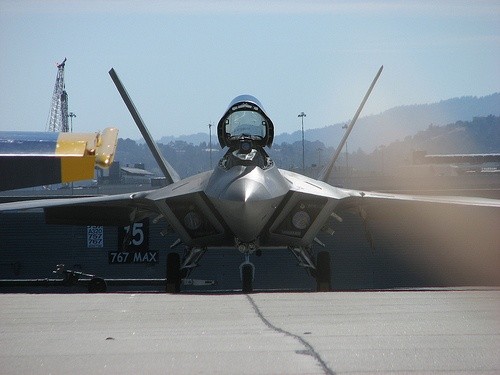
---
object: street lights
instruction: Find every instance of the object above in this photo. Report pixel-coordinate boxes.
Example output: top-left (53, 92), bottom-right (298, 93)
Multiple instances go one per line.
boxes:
top-left (206, 122), bottom-right (214, 170)
top-left (297, 112), bottom-right (307, 173)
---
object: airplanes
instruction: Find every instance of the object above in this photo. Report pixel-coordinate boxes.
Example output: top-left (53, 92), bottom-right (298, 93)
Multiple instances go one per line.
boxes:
top-left (0, 65), bottom-right (499, 293)
